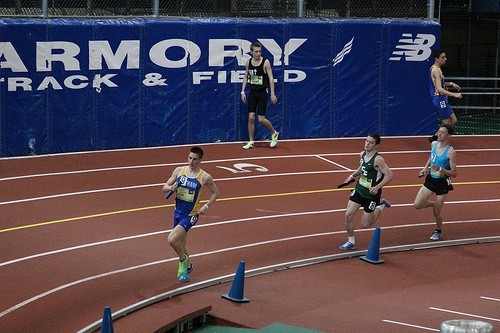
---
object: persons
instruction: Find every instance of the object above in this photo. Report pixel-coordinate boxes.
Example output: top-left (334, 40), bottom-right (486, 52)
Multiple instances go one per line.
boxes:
top-left (428, 50), bottom-right (463, 145)
top-left (241, 42), bottom-right (280, 150)
top-left (414, 124), bottom-right (458, 241)
top-left (162, 147), bottom-right (221, 282)
top-left (339, 133), bottom-right (393, 252)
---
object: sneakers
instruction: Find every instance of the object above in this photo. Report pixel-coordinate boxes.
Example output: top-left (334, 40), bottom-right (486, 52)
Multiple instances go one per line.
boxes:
top-left (178, 265), bottom-right (194, 282)
top-left (176, 256), bottom-right (189, 280)
top-left (429, 135), bottom-right (437, 142)
top-left (338, 241), bottom-right (356, 251)
top-left (380, 198), bottom-right (391, 209)
top-left (242, 141), bottom-right (256, 150)
top-left (430, 229), bottom-right (441, 241)
top-left (270, 133), bottom-right (280, 148)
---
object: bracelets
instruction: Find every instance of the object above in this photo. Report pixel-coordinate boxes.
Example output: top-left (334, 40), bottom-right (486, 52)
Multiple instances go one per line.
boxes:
top-left (439, 166), bottom-right (442, 172)
top-left (241, 92), bottom-right (245, 95)
top-left (204, 204), bottom-right (209, 210)
top-left (350, 175), bottom-right (354, 179)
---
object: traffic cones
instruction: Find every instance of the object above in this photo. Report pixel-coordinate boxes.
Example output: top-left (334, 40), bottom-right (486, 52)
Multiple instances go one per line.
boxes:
top-left (221, 261), bottom-right (251, 304)
top-left (358, 227), bottom-right (385, 265)
top-left (100, 306), bottom-right (116, 333)
